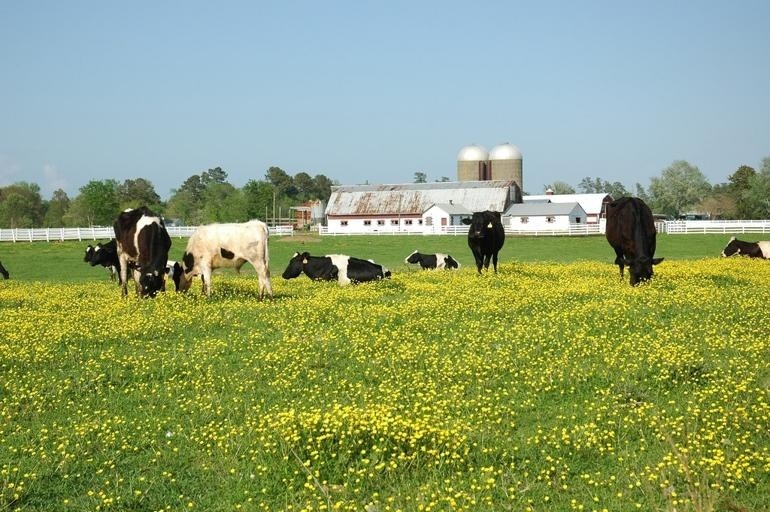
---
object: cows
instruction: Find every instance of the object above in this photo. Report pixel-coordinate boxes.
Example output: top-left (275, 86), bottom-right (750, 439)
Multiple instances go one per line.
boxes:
top-left (605, 196), bottom-right (665, 288)
top-left (0, 261), bottom-right (9, 279)
top-left (281, 250), bottom-right (392, 287)
top-left (403, 249), bottom-right (460, 271)
top-left (720, 235), bottom-right (770, 261)
top-left (171, 218), bottom-right (274, 301)
top-left (460, 210), bottom-right (506, 275)
top-left (83, 205), bottom-right (178, 300)
